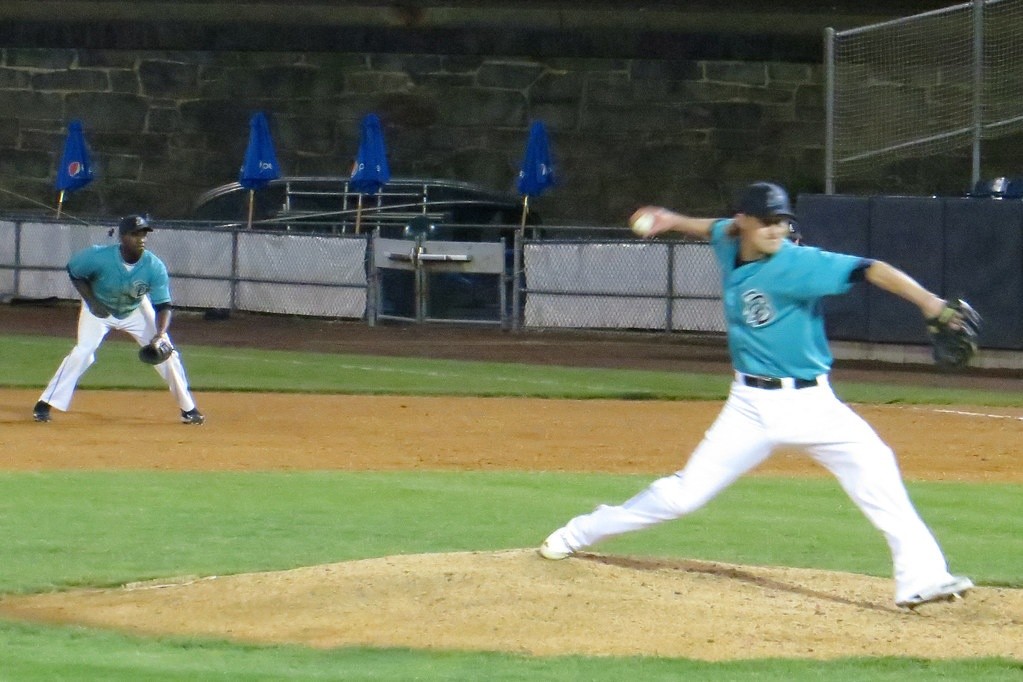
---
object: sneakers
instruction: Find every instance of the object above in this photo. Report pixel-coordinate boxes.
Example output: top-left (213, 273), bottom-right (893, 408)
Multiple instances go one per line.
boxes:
top-left (540, 527), bottom-right (576, 559)
top-left (182, 409), bottom-right (204, 424)
top-left (897, 576), bottom-right (975, 606)
top-left (32, 405), bottom-right (51, 423)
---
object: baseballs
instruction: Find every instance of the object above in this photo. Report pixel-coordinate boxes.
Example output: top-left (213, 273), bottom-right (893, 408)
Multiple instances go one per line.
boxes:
top-left (632, 215), bottom-right (657, 238)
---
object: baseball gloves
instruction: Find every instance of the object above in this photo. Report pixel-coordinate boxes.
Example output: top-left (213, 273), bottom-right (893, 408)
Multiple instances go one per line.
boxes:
top-left (931, 300), bottom-right (982, 368)
top-left (138, 343), bottom-right (172, 365)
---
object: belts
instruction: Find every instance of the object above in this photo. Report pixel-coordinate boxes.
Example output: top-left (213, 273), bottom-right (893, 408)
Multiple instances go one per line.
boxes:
top-left (744, 370), bottom-right (821, 389)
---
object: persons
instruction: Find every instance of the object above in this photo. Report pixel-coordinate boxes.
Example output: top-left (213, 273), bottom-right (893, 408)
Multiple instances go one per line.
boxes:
top-left (537, 180), bottom-right (987, 611)
top-left (30, 215), bottom-right (206, 427)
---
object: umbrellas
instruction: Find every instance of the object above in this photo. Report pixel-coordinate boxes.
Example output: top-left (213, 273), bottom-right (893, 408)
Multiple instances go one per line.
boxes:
top-left (52, 116), bottom-right (96, 223)
top-left (236, 109), bottom-right (282, 232)
top-left (347, 108), bottom-right (392, 236)
top-left (514, 117), bottom-right (561, 241)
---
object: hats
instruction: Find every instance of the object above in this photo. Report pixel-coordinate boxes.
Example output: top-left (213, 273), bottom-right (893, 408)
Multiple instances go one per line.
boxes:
top-left (736, 181), bottom-right (798, 222)
top-left (120, 215), bottom-right (154, 233)
